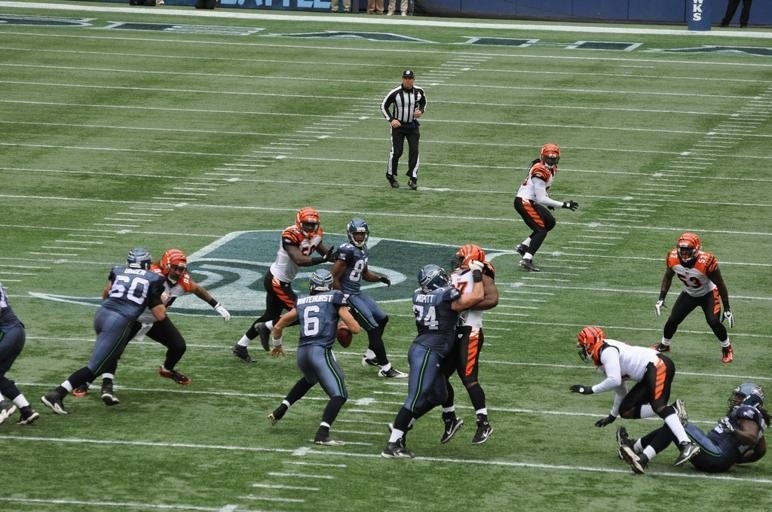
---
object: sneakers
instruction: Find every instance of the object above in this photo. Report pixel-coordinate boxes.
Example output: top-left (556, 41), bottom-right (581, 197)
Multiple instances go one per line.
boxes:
top-left (671, 398), bottom-right (688, 428)
top-left (344, 8), bottom-right (349, 12)
top-left (386, 11), bottom-right (394, 16)
top-left (471, 413), bottom-right (494, 445)
top-left (267, 412), bottom-right (279, 426)
top-left (100, 389), bottom-right (119, 406)
top-left (647, 342), bottom-right (670, 352)
top-left (378, 362), bottom-right (408, 378)
top-left (518, 258), bottom-right (540, 272)
top-left (361, 353), bottom-right (378, 368)
top-left (388, 421), bottom-right (413, 447)
top-left (721, 345), bottom-right (733, 363)
top-left (386, 173), bottom-right (400, 188)
top-left (40, 390), bottom-right (69, 415)
top-left (673, 441), bottom-right (700, 467)
top-left (159, 367), bottom-right (192, 385)
top-left (71, 388), bottom-right (87, 397)
top-left (314, 432), bottom-right (344, 446)
top-left (401, 12), bottom-right (406, 16)
top-left (381, 438), bottom-right (415, 459)
top-left (254, 322), bottom-right (271, 351)
top-left (230, 343), bottom-right (257, 363)
top-left (440, 412), bottom-right (463, 444)
top-left (15, 405), bottom-right (39, 425)
top-left (619, 445), bottom-right (649, 475)
top-left (616, 425), bottom-right (636, 460)
top-left (514, 242), bottom-right (528, 256)
top-left (408, 178), bottom-right (417, 190)
top-left (330, 8), bottom-right (338, 12)
top-left (0, 400), bottom-right (17, 426)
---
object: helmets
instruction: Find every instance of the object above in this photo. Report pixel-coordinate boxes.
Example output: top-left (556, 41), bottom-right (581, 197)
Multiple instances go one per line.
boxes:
top-left (160, 249), bottom-right (187, 280)
top-left (677, 232), bottom-right (700, 263)
top-left (346, 218), bottom-right (369, 248)
top-left (539, 143), bottom-right (560, 169)
top-left (451, 244), bottom-right (485, 275)
top-left (576, 325), bottom-right (605, 366)
top-left (418, 263), bottom-right (452, 294)
top-left (308, 268), bottom-right (334, 295)
top-left (726, 383), bottom-right (764, 409)
top-left (295, 207), bottom-right (320, 238)
top-left (126, 247), bottom-right (152, 270)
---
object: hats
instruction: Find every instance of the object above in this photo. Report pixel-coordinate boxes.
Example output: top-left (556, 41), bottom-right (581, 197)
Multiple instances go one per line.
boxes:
top-left (402, 70), bottom-right (414, 77)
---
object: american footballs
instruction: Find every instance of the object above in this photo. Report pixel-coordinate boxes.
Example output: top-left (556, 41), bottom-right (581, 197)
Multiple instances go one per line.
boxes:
top-left (336, 328), bottom-right (352, 348)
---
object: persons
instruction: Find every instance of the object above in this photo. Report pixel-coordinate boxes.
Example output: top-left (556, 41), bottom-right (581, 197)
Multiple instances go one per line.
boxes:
top-left (616, 382), bottom-right (771, 474)
top-left (380, 70), bottom-right (427, 189)
top-left (101, 248), bottom-right (231, 406)
top-left (381, 259), bottom-right (484, 458)
top-left (267, 269), bottom-right (360, 445)
top-left (41, 247), bottom-right (166, 416)
top-left (387, 0), bottom-right (408, 17)
top-left (331, 0), bottom-right (351, 13)
top-left (230, 207), bottom-right (335, 362)
top-left (367, 0), bottom-right (384, 16)
top-left (654, 233), bottom-right (735, 364)
top-left (330, 217), bottom-right (409, 379)
top-left (569, 325), bottom-right (700, 467)
top-left (439, 244), bottom-right (498, 444)
top-left (514, 144), bottom-right (578, 272)
top-left (0, 281), bottom-right (40, 425)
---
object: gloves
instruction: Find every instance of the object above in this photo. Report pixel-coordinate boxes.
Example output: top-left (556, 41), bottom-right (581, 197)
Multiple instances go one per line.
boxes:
top-left (562, 200), bottom-right (579, 211)
top-left (721, 311), bottom-right (735, 328)
top-left (569, 384), bottom-right (594, 395)
top-left (321, 245), bottom-right (334, 262)
top-left (594, 413), bottom-right (616, 428)
top-left (654, 300), bottom-right (668, 318)
top-left (378, 276), bottom-right (391, 288)
top-left (468, 259), bottom-right (484, 273)
top-left (213, 302), bottom-right (231, 322)
